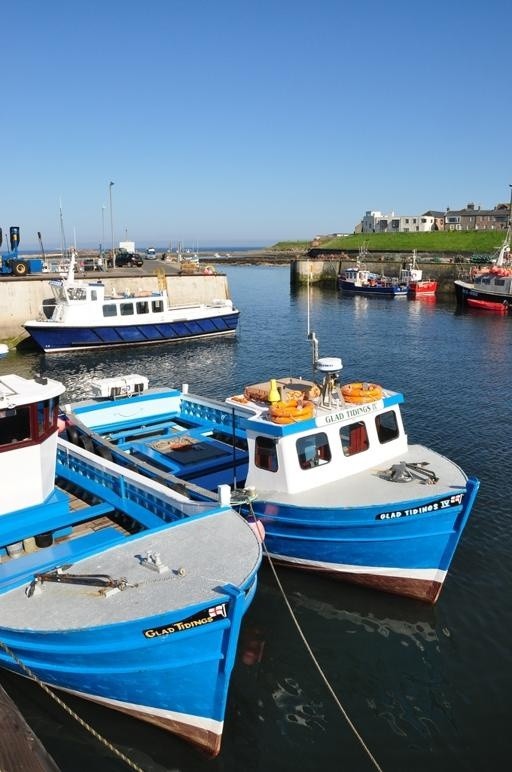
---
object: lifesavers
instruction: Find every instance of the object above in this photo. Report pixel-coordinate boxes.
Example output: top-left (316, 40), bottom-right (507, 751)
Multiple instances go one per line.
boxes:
top-left (341, 382), bottom-right (382, 403)
top-left (269, 399), bottom-right (314, 424)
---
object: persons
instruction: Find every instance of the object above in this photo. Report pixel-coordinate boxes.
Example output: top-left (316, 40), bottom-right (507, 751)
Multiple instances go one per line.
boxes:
top-left (97, 277), bottom-right (102, 283)
top-left (203, 266), bottom-right (213, 275)
top-left (280, 374), bottom-right (377, 417)
top-left (95, 254), bottom-right (113, 273)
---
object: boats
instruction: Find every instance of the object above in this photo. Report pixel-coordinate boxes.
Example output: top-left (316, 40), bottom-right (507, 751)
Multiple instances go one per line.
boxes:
top-left (454, 184), bottom-right (511, 310)
top-left (0, 227), bottom-right (47, 276)
top-left (60, 257), bottom-right (480, 606)
top-left (0, 373), bottom-right (266, 760)
top-left (338, 237), bottom-right (440, 297)
top-left (108, 253), bottom-right (144, 267)
top-left (146, 248), bottom-right (157, 260)
top-left (19, 247), bottom-right (240, 355)
top-left (100, 182), bottom-right (115, 269)
